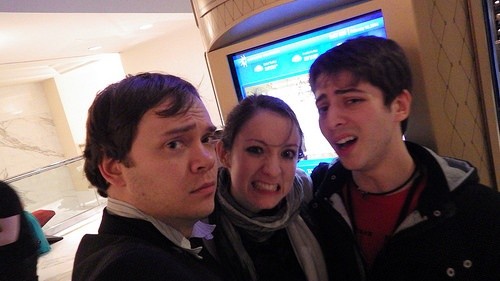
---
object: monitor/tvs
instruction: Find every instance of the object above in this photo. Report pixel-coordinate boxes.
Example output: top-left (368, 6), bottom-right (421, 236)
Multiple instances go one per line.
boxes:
top-left (205, 1), bottom-right (406, 175)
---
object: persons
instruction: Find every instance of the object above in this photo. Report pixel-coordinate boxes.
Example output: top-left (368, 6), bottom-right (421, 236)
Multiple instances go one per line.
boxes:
top-left (72, 72), bottom-right (221, 281)
top-left (0, 181), bottom-right (40, 281)
top-left (188, 95), bottom-right (352, 281)
top-left (309, 36), bottom-right (500, 281)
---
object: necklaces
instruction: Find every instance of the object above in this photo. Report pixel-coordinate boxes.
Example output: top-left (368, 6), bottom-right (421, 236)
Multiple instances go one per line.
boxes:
top-left (352, 163), bottom-right (420, 200)
